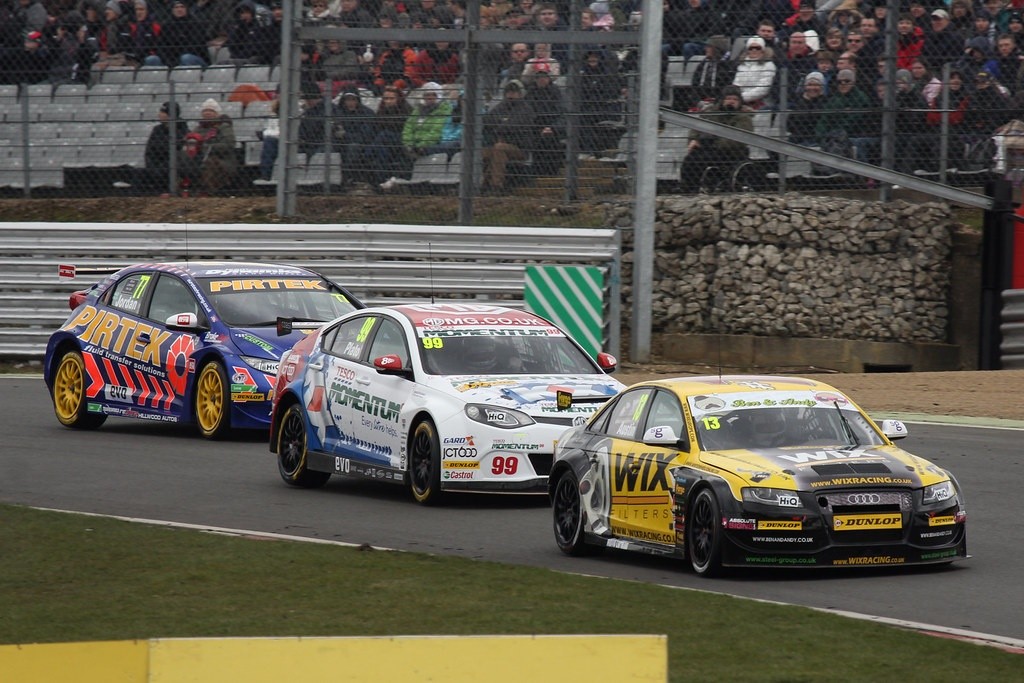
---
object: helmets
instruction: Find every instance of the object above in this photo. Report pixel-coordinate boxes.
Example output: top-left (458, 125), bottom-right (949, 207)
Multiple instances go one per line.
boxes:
top-left (461, 337), bottom-right (497, 371)
top-left (750, 410), bottom-right (787, 446)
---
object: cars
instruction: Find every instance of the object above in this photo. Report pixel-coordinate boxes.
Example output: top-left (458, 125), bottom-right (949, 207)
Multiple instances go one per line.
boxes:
top-left (269, 302), bottom-right (649, 508)
top-left (44, 261), bottom-right (403, 440)
top-left (547, 375), bottom-right (972, 578)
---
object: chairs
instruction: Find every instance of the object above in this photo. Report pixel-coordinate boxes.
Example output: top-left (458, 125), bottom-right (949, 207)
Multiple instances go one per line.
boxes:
top-left (618, 35), bottom-right (857, 180)
top-left (0, 64), bottom-right (483, 194)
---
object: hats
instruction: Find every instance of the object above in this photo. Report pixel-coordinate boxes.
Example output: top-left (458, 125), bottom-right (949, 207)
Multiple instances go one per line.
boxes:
top-left (745, 35), bottom-right (766, 49)
top-left (721, 85), bottom-right (743, 100)
top-left (896, 69), bottom-right (912, 84)
top-left (160, 102), bottom-right (180, 118)
top-left (804, 72), bottom-right (825, 88)
top-left (972, 71), bottom-right (989, 83)
top-left (343, 88), bottom-right (359, 101)
top-left (199, 98), bottom-right (222, 116)
top-left (700, 35), bottom-right (731, 55)
top-left (931, 8), bottom-right (950, 21)
top-left (505, 83), bottom-right (518, 91)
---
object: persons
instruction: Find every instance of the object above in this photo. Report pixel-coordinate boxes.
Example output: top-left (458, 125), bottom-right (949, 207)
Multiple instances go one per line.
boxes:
top-left (0, 0), bottom-right (282, 86)
top-left (465, 336), bottom-right (500, 374)
top-left (747, 409), bottom-right (788, 449)
top-left (255, 0), bottom-right (1024, 194)
top-left (181, 98), bottom-right (241, 197)
top-left (130, 99), bottom-right (191, 197)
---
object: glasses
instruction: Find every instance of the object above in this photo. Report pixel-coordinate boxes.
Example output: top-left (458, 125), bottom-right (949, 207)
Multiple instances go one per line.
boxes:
top-left (747, 46), bottom-right (763, 51)
top-left (835, 79), bottom-right (852, 85)
top-left (847, 39), bottom-right (864, 45)
